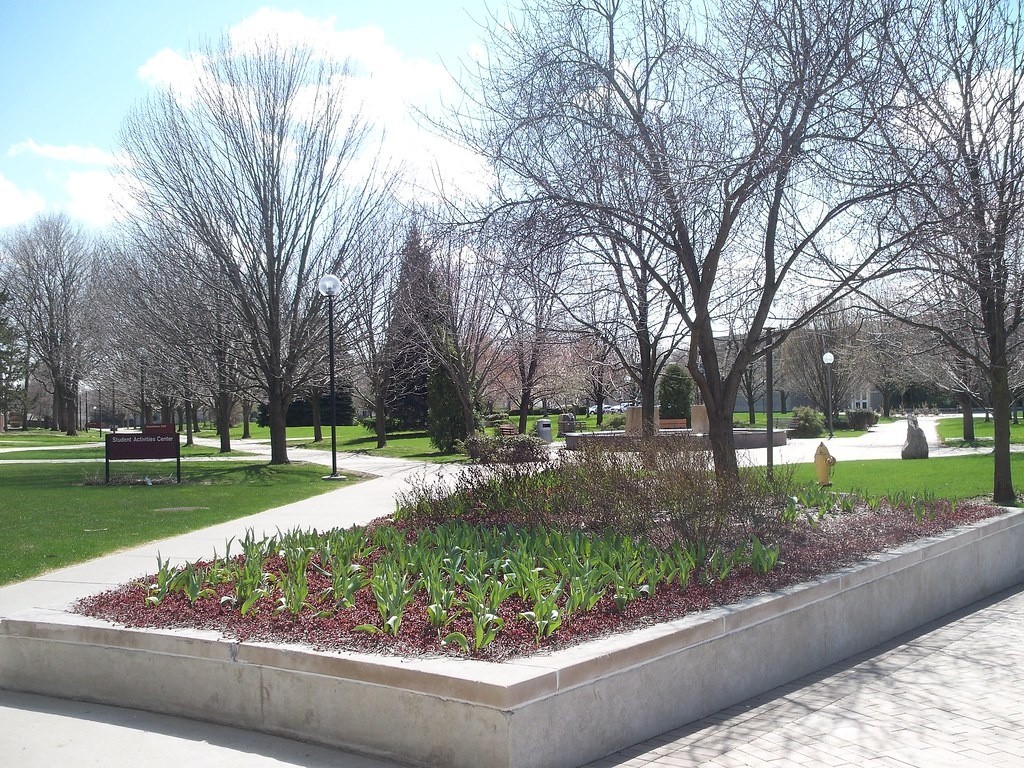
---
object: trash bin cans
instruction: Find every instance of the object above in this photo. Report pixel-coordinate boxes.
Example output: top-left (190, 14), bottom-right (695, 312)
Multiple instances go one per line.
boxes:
top-left (110, 425), bottom-right (118, 431)
top-left (538, 419), bottom-right (552, 444)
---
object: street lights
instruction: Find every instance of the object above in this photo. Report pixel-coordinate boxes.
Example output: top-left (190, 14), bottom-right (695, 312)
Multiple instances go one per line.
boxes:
top-left (136, 346), bottom-right (147, 430)
top-left (318, 273), bottom-right (341, 478)
top-left (822, 351), bottom-right (834, 436)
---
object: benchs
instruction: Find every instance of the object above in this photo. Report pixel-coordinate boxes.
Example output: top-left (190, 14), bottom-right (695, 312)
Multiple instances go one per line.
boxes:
top-left (660, 419), bottom-right (686, 429)
top-left (772, 417), bottom-right (799, 440)
top-left (558, 421), bottom-right (587, 438)
top-left (500, 424), bottom-right (518, 437)
